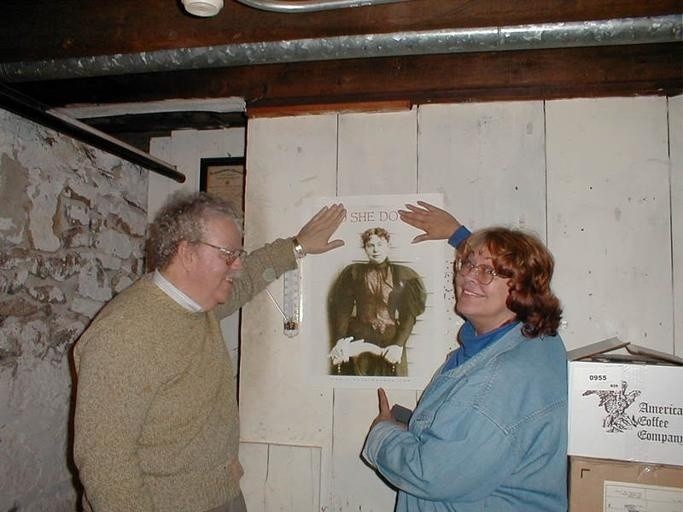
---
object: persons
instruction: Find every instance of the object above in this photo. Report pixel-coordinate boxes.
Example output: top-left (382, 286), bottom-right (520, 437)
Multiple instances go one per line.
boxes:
top-left (357, 197), bottom-right (570, 511)
top-left (325, 226), bottom-right (427, 376)
top-left (66, 187), bottom-right (348, 509)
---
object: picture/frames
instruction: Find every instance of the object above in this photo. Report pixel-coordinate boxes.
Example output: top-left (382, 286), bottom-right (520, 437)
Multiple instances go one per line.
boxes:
top-left (200, 156), bottom-right (244, 222)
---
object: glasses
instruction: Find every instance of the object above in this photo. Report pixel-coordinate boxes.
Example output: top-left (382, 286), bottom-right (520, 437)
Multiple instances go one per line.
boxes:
top-left (191, 237), bottom-right (250, 269)
top-left (453, 256), bottom-right (515, 288)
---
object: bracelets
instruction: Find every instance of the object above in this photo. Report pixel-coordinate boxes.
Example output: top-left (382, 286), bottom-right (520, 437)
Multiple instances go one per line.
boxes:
top-left (289, 234), bottom-right (308, 260)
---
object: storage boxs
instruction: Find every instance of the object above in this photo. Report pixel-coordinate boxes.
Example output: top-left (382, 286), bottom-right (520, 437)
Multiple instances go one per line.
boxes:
top-left (564, 336), bottom-right (683, 507)
